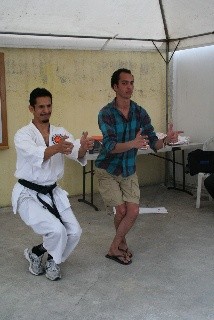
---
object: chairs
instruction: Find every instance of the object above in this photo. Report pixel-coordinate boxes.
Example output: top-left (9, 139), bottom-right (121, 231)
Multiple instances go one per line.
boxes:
top-left (195, 136), bottom-right (214, 210)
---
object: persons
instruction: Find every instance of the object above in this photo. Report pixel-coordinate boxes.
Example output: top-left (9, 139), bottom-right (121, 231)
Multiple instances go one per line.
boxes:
top-left (11, 87), bottom-right (94, 281)
top-left (94, 69), bottom-right (184, 265)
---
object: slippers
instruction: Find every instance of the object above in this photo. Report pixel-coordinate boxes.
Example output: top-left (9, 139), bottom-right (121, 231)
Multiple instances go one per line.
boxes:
top-left (106, 254), bottom-right (132, 264)
top-left (120, 248), bottom-right (132, 257)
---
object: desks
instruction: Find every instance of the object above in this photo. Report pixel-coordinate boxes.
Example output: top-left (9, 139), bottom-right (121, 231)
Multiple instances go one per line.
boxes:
top-left (71, 133), bottom-right (205, 217)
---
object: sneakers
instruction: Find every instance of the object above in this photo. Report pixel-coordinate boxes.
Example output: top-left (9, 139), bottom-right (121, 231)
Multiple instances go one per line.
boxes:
top-left (24, 247), bottom-right (44, 276)
top-left (45, 259), bottom-right (62, 280)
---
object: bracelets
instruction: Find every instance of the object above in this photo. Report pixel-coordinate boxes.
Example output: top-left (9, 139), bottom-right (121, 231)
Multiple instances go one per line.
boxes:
top-left (163, 136), bottom-right (169, 145)
